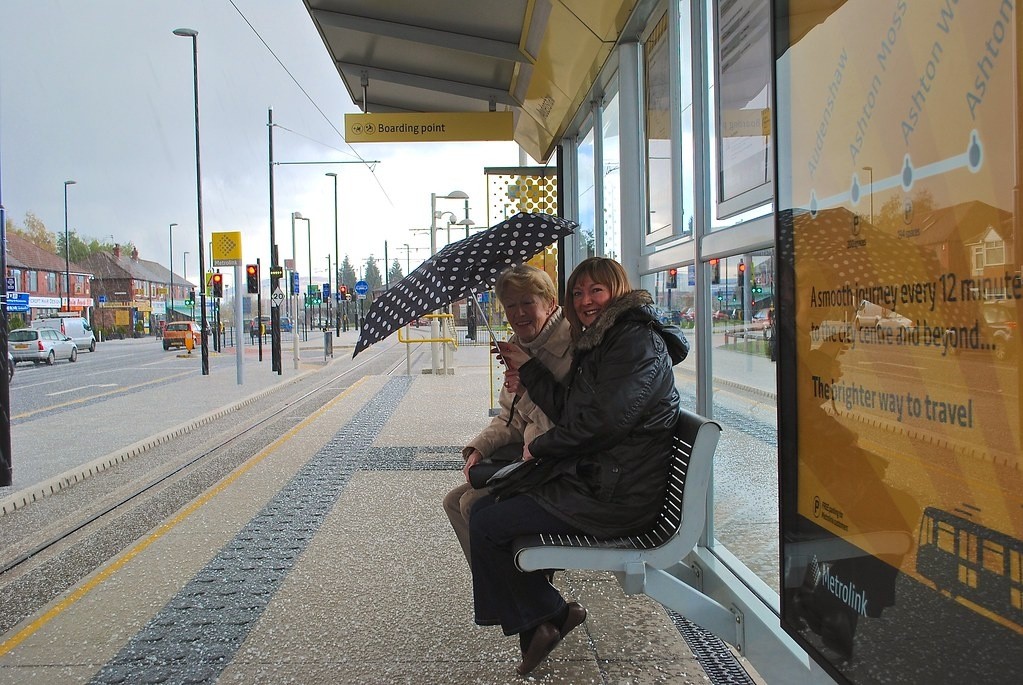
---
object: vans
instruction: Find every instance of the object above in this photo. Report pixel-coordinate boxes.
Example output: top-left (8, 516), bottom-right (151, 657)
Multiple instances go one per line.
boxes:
top-left (250, 315), bottom-right (273, 336)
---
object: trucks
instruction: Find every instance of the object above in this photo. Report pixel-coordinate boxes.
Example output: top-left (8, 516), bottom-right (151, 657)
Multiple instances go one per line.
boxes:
top-left (31, 311), bottom-right (97, 352)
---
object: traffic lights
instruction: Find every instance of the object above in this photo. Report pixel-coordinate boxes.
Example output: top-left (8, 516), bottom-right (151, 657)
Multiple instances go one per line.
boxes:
top-left (709, 259), bottom-right (721, 285)
top-left (752, 287), bottom-right (763, 293)
top-left (311, 297), bottom-right (322, 305)
top-left (212, 274), bottom-right (222, 298)
top-left (304, 303), bottom-right (309, 307)
top-left (245, 264), bottom-right (259, 293)
top-left (185, 291), bottom-right (196, 305)
top-left (666, 268), bottom-right (678, 289)
top-left (717, 289), bottom-right (723, 301)
top-left (752, 296), bottom-right (756, 306)
top-left (736, 262), bottom-right (745, 287)
top-left (733, 293), bottom-right (737, 302)
top-left (340, 286), bottom-right (347, 300)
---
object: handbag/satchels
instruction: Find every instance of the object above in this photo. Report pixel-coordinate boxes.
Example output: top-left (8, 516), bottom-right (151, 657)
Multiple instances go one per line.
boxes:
top-left (486, 458), bottom-right (541, 494)
top-left (468, 442), bottom-right (523, 489)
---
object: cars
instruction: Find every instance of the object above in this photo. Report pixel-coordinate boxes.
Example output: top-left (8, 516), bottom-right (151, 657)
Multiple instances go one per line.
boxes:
top-left (656, 296), bottom-right (775, 328)
top-left (7, 326), bottom-right (78, 366)
top-left (162, 321), bottom-right (201, 350)
top-left (856, 299), bottom-right (1020, 358)
top-left (280, 317), bottom-right (291, 332)
top-left (242, 319), bottom-right (253, 333)
top-left (311, 316), bottom-right (336, 328)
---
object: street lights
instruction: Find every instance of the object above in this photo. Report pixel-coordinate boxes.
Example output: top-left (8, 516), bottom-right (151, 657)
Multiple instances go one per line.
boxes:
top-left (442, 219), bottom-right (475, 375)
top-left (429, 188), bottom-right (470, 375)
top-left (172, 27), bottom-right (214, 376)
top-left (183, 251), bottom-right (190, 289)
top-left (403, 243), bottom-right (410, 274)
top-left (63, 180), bottom-right (77, 311)
top-left (295, 216), bottom-right (314, 331)
top-left (169, 223), bottom-right (178, 322)
top-left (325, 172), bottom-right (339, 337)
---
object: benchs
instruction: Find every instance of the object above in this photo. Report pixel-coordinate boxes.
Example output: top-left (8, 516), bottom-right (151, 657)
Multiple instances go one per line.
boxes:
top-left (511, 409), bottom-right (745, 656)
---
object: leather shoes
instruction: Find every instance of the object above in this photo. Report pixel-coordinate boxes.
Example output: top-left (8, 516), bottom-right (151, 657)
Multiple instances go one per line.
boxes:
top-left (560, 602), bottom-right (587, 640)
top-left (516, 622), bottom-right (560, 675)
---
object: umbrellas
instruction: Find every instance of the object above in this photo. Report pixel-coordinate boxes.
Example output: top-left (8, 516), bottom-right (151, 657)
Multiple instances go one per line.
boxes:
top-left (352, 212), bottom-right (580, 372)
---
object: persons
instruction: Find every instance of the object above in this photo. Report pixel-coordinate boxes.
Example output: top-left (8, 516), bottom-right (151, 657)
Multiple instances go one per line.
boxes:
top-left (469, 257), bottom-right (690, 674)
top-left (442, 265), bottom-right (586, 579)
